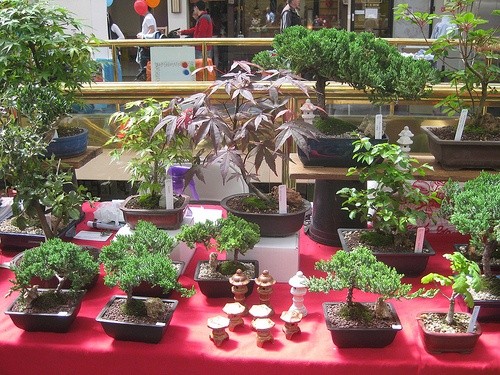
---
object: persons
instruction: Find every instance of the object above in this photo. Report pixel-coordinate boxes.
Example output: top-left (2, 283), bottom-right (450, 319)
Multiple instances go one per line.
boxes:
top-left (266, 7), bottom-right (275, 23)
top-left (136, 8), bottom-right (157, 81)
top-left (280, 0), bottom-right (303, 32)
top-left (178, 0), bottom-right (213, 59)
top-left (107, 12), bottom-right (126, 82)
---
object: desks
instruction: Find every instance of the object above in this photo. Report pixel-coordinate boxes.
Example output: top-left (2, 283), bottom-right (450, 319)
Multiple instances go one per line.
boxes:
top-left (44, 145), bottom-right (102, 191)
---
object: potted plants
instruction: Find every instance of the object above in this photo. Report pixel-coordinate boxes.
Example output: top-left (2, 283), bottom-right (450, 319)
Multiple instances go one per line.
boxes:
top-left (304, 242), bottom-right (440, 350)
top-left (0, 0), bottom-right (102, 292)
top-left (251, 24), bottom-right (442, 171)
top-left (93, 220), bottom-right (197, 344)
top-left (335, 129), bottom-right (443, 276)
top-left (4, 234), bottom-right (100, 335)
top-left (415, 250), bottom-right (494, 355)
top-left (103, 98), bottom-right (215, 229)
top-left (149, 59), bottom-right (330, 238)
top-left (173, 212), bottom-right (262, 299)
top-left (392, 0), bottom-right (500, 167)
top-left (435, 169), bottom-right (500, 321)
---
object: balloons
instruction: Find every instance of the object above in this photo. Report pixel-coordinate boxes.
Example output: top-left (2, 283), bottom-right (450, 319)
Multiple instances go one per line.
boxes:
top-left (134, 0), bottom-right (148, 16)
top-left (146, 0), bottom-right (160, 10)
top-left (107, 0), bottom-right (113, 7)
